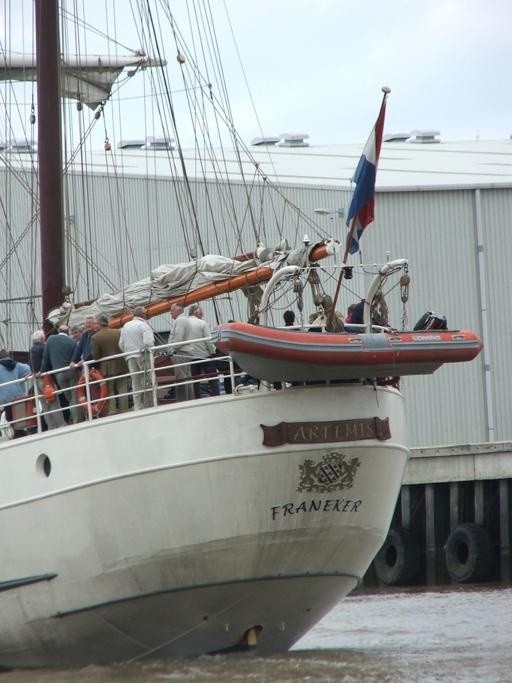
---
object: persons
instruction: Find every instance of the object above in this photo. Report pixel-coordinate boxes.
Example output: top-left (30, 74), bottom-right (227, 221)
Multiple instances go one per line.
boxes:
top-left (308, 297), bottom-right (385, 333)
top-left (187, 304), bottom-right (220, 400)
top-left (31, 325), bottom-right (86, 429)
top-left (165, 302), bottom-right (195, 403)
top-left (216, 320), bottom-right (242, 393)
top-left (120, 307), bottom-right (155, 411)
top-left (283, 311), bottom-right (301, 332)
top-left (1, 348), bottom-right (34, 439)
top-left (70, 315), bottom-right (131, 416)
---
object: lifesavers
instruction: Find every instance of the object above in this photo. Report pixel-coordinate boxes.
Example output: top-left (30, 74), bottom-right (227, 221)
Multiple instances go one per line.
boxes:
top-left (77, 370), bottom-right (108, 415)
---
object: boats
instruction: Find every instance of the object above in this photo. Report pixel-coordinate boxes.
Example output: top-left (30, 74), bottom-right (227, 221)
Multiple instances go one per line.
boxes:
top-left (212, 312), bottom-right (483, 382)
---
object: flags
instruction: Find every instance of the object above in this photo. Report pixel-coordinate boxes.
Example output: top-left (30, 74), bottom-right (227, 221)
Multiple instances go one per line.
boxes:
top-left (343, 94), bottom-right (387, 257)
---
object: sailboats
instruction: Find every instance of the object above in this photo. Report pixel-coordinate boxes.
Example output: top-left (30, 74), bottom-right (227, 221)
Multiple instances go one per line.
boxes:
top-left (0, 0), bottom-right (410, 667)
